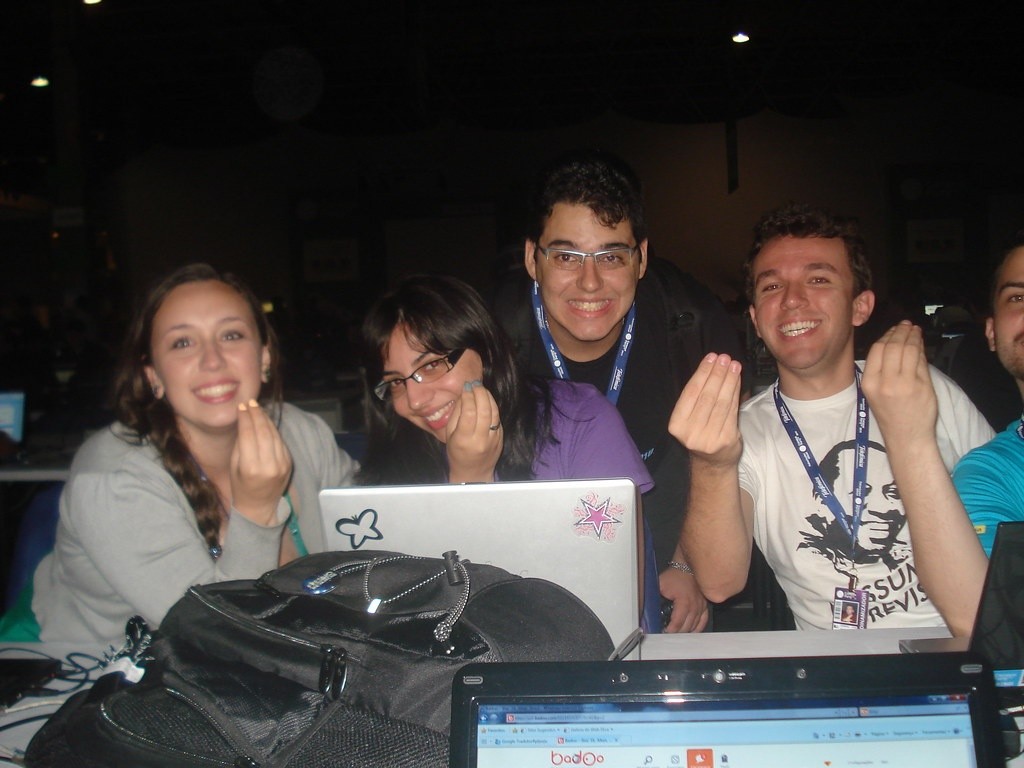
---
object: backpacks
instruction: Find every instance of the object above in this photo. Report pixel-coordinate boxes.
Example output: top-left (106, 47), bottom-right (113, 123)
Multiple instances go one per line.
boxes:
top-left (23, 550), bottom-right (646, 768)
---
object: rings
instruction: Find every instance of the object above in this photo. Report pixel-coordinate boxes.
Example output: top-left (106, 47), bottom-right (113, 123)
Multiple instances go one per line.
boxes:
top-left (489, 423), bottom-right (501, 430)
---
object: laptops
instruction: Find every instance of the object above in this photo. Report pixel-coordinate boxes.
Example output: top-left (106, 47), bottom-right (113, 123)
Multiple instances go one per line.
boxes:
top-left (0, 391), bottom-right (28, 448)
top-left (318, 477), bottom-right (643, 664)
top-left (451, 649), bottom-right (1005, 768)
top-left (898, 522), bottom-right (1024, 710)
top-left (0, 658), bottom-right (63, 710)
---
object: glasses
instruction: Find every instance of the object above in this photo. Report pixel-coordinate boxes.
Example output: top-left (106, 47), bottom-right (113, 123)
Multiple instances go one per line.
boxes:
top-left (372, 347), bottom-right (469, 402)
top-left (537, 244), bottom-right (638, 272)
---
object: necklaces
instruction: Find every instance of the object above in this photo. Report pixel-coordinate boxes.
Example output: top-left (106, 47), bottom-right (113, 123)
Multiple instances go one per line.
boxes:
top-left (188, 455), bottom-right (231, 522)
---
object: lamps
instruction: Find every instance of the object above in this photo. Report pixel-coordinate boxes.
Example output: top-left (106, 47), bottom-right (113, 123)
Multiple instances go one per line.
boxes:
top-left (30, 63), bottom-right (49, 87)
top-left (733, 24), bottom-right (749, 43)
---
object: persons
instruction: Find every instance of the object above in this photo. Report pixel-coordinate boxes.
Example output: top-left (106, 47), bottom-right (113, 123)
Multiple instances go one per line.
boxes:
top-left (482, 157), bottom-right (752, 634)
top-left (33, 263), bottom-right (360, 647)
top-left (842, 605), bottom-right (856, 623)
top-left (361, 272), bottom-right (655, 495)
top-left (668, 200), bottom-right (998, 632)
top-left (861, 242), bottom-right (1024, 638)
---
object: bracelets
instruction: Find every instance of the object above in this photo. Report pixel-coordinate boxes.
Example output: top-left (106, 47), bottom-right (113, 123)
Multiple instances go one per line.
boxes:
top-left (668, 561), bottom-right (692, 573)
